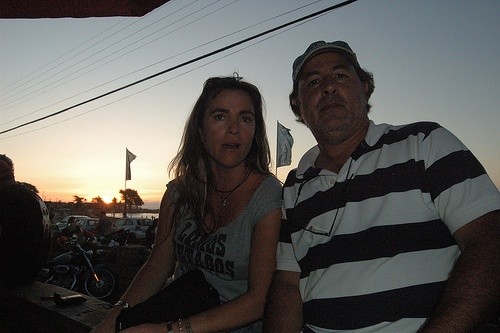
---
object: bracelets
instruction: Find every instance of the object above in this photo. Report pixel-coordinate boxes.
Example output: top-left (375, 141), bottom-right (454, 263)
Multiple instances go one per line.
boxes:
top-left (167, 321), bottom-right (173, 333)
top-left (112, 300), bottom-right (131, 309)
top-left (182, 319), bottom-right (193, 333)
top-left (178, 319), bottom-right (184, 333)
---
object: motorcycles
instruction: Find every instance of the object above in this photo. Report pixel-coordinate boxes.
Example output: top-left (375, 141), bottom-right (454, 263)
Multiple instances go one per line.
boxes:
top-left (40, 232), bottom-right (118, 300)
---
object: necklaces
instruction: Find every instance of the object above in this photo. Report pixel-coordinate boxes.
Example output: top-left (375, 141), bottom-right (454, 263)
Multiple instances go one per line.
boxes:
top-left (210, 167), bottom-right (253, 206)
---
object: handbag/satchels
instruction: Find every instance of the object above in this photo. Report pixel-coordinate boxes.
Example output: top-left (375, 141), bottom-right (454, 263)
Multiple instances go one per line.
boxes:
top-left (115, 269), bottom-right (219, 333)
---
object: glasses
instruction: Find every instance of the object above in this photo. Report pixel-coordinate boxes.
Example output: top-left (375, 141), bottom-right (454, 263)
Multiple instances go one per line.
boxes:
top-left (286, 174), bottom-right (353, 235)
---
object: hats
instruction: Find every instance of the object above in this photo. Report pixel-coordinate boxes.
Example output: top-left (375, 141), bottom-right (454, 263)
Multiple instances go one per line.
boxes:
top-left (292, 39), bottom-right (356, 84)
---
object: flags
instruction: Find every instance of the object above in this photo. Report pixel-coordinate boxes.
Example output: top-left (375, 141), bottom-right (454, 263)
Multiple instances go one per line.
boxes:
top-left (126, 148), bottom-right (136, 180)
top-left (276, 120), bottom-right (294, 167)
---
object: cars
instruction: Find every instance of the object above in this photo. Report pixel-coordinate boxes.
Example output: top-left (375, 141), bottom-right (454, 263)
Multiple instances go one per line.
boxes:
top-left (54, 213), bottom-right (88, 230)
top-left (76, 218), bottom-right (99, 231)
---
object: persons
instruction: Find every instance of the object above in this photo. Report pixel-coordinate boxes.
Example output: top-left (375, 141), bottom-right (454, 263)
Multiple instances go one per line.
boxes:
top-left (88, 72), bottom-right (283, 333)
top-left (95, 211), bottom-right (112, 235)
top-left (263, 42), bottom-right (500, 333)
top-left (0, 155), bottom-right (64, 270)
top-left (60, 217), bottom-right (81, 236)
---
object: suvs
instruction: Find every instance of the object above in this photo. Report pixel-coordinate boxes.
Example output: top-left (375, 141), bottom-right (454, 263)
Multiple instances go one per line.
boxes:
top-left (110, 216), bottom-right (152, 243)
